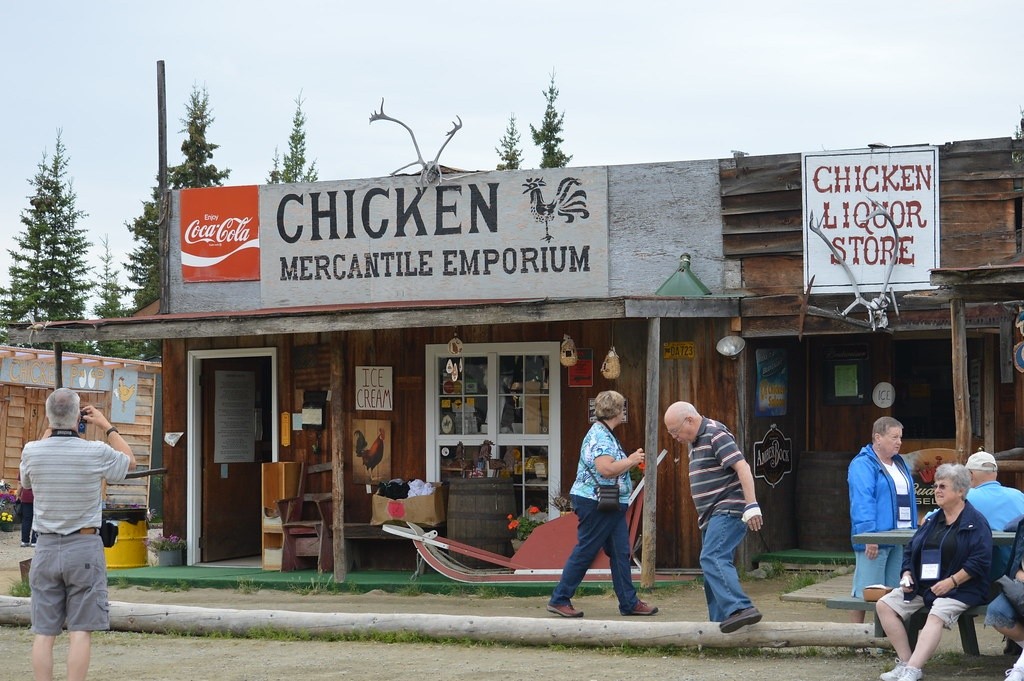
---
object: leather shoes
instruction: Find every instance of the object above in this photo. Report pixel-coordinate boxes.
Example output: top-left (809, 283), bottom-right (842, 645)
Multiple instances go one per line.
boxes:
top-left (719, 606), bottom-right (762, 633)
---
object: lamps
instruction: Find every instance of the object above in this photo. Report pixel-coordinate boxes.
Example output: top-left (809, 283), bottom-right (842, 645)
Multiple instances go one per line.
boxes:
top-left (655, 253), bottom-right (712, 296)
top-left (716, 336), bottom-right (746, 361)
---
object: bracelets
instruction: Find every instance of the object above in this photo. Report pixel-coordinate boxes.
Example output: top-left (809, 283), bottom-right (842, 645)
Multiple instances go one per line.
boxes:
top-left (951, 575), bottom-right (957, 588)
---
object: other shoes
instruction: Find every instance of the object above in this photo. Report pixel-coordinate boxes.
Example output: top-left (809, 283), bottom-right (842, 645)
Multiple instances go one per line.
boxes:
top-left (21, 542), bottom-right (36, 547)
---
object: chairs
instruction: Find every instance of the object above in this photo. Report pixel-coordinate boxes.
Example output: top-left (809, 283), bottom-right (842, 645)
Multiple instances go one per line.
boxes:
top-left (273, 461), bottom-right (335, 574)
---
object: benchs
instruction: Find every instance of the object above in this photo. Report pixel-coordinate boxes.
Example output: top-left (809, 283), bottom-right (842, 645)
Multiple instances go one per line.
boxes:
top-left (824, 596), bottom-right (991, 655)
top-left (329, 524), bottom-right (429, 575)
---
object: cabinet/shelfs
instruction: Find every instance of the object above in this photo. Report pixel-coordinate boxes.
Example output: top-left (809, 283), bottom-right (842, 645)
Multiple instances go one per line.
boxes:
top-left (261, 462), bottom-right (298, 572)
top-left (437, 389), bottom-right (550, 491)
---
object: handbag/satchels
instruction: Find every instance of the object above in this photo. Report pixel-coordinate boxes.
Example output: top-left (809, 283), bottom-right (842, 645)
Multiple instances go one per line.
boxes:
top-left (14, 498), bottom-right (22, 516)
top-left (597, 485), bottom-right (620, 512)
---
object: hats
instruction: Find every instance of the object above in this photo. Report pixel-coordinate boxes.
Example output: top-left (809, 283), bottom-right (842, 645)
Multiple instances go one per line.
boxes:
top-left (964, 451), bottom-right (998, 471)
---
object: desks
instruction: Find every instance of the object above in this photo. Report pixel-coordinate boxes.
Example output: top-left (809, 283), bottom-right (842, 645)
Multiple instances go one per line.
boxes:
top-left (852, 528), bottom-right (1018, 655)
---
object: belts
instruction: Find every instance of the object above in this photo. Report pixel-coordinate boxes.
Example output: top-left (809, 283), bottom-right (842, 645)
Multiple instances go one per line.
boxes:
top-left (41, 527), bottom-right (97, 535)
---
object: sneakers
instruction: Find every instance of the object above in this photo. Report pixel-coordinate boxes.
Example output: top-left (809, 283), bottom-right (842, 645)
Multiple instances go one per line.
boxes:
top-left (547, 602), bottom-right (583, 617)
top-left (880, 662), bottom-right (908, 681)
top-left (621, 601), bottom-right (658, 616)
top-left (896, 666), bottom-right (923, 681)
top-left (1005, 664), bottom-right (1024, 681)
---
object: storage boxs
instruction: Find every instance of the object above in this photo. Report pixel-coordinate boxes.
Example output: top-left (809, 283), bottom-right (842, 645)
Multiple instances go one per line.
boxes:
top-left (863, 586), bottom-right (892, 602)
top-left (369, 482), bottom-right (445, 526)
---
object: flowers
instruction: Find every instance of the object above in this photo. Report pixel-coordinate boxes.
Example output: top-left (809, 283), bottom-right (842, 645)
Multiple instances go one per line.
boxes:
top-left (506, 504), bottom-right (545, 542)
top-left (629, 461), bottom-right (646, 481)
top-left (141, 532), bottom-right (187, 558)
top-left (0, 479), bottom-right (18, 524)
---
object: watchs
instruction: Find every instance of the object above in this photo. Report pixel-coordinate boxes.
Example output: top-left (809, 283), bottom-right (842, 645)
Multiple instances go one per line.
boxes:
top-left (106, 426), bottom-right (119, 436)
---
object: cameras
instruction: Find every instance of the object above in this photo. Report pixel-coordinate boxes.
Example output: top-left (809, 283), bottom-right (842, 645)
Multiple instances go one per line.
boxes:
top-left (79, 411), bottom-right (87, 432)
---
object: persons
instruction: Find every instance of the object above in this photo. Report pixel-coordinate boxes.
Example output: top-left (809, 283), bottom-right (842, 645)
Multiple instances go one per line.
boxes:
top-left (547, 391), bottom-right (658, 620)
top-left (846, 417), bottom-right (917, 655)
top-left (985, 517), bottom-right (1024, 681)
top-left (664, 400), bottom-right (762, 633)
top-left (921, 451), bottom-right (1024, 656)
top-left (875, 462), bottom-right (993, 681)
top-left (20, 389), bottom-right (136, 681)
top-left (18, 473), bottom-right (38, 547)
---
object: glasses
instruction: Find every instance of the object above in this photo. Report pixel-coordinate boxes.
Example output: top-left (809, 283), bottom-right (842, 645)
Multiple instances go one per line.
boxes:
top-left (668, 418), bottom-right (687, 435)
top-left (933, 482), bottom-right (948, 491)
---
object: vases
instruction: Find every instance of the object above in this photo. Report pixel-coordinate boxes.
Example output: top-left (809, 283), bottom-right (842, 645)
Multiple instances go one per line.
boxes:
top-left (147, 549), bottom-right (183, 567)
top-left (511, 539), bottom-right (524, 554)
top-left (1, 521), bottom-right (16, 532)
top-left (631, 479), bottom-right (639, 489)
